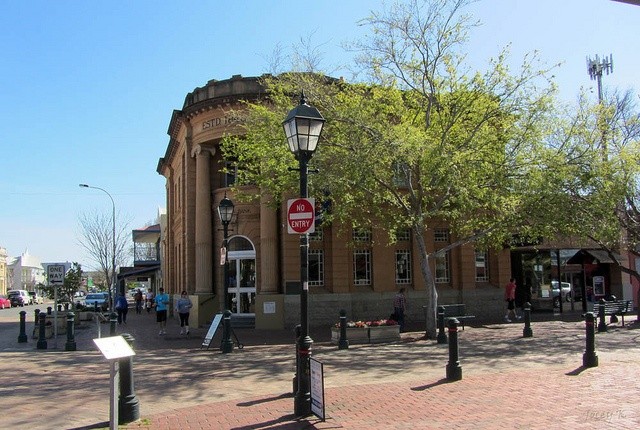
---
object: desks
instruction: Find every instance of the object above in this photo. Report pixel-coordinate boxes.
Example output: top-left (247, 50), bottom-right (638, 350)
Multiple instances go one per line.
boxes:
top-left (78, 184), bottom-right (115, 325)
top-left (217, 191), bottom-right (236, 354)
top-left (283, 91), bottom-right (327, 417)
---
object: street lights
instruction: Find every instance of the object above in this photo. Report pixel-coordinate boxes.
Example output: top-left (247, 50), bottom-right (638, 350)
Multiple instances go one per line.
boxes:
top-left (115, 301), bottom-right (122, 310)
top-left (389, 314), bottom-right (397, 321)
top-left (146, 302), bottom-right (151, 308)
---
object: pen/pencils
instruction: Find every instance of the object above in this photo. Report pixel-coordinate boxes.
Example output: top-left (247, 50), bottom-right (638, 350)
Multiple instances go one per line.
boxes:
top-left (9, 290), bottom-right (33, 307)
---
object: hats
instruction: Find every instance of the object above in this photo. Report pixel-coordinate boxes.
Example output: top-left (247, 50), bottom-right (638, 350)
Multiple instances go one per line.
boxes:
top-left (28, 292), bottom-right (43, 304)
top-left (84, 293), bottom-right (107, 311)
top-left (0, 295), bottom-right (11, 310)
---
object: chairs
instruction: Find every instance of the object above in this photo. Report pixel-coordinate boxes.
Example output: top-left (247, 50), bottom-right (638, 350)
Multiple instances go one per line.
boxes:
top-left (163, 331), bottom-right (167, 335)
top-left (159, 331), bottom-right (163, 336)
top-left (505, 319), bottom-right (511, 323)
top-left (186, 331), bottom-right (190, 334)
top-left (516, 316), bottom-right (521, 320)
top-left (180, 331), bottom-right (183, 334)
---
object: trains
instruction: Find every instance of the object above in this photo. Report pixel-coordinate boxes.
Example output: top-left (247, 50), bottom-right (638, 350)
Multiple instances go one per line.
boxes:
top-left (288, 199), bottom-right (314, 234)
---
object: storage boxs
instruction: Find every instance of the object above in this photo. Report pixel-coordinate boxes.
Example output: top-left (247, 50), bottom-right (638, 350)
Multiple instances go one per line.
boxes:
top-left (581, 301), bottom-right (634, 328)
top-left (444, 304), bottom-right (476, 330)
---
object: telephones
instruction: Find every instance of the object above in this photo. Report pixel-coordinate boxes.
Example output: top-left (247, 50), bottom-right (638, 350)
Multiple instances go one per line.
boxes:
top-left (48, 265), bottom-right (64, 285)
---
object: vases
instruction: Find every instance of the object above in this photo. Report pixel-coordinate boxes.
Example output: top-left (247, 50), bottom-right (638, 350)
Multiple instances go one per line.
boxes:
top-left (333, 317), bottom-right (399, 327)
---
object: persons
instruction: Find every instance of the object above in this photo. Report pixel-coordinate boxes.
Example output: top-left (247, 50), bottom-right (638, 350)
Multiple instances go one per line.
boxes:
top-left (146, 288), bottom-right (154, 306)
top-left (135, 288), bottom-right (143, 315)
top-left (394, 287), bottom-right (407, 333)
top-left (155, 287), bottom-right (170, 336)
top-left (505, 278), bottom-right (522, 323)
top-left (147, 300), bottom-right (151, 312)
top-left (177, 291), bottom-right (193, 335)
top-left (115, 291), bottom-right (128, 325)
